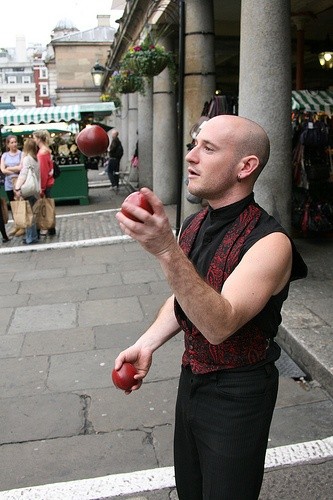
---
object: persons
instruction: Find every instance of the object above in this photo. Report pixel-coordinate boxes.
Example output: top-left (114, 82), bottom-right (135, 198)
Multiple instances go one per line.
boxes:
top-left (0, 127), bottom-right (58, 246)
top-left (113, 115), bottom-right (309, 500)
top-left (107, 131), bottom-right (124, 190)
top-left (130, 129), bottom-right (139, 188)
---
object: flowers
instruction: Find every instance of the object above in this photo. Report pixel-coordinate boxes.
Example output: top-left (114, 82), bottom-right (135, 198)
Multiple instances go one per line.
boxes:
top-left (100, 93), bottom-right (117, 101)
top-left (109, 70), bottom-right (148, 95)
top-left (125, 35), bottom-right (168, 57)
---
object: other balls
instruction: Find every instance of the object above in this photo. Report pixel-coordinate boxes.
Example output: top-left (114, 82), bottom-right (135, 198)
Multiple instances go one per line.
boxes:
top-left (78, 126), bottom-right (109, 158)
top-left (112, 363), bottom-right (138, 391)
top-left (121, 191), bottom-right (154, 223)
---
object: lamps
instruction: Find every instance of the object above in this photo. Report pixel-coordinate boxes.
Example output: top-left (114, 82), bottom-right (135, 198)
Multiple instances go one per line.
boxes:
top-left (91, 60), bottom-right (110, 86)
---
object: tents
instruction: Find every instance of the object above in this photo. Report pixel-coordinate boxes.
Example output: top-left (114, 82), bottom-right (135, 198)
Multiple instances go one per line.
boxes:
top-left (0, 101), bottom-right (116, 126)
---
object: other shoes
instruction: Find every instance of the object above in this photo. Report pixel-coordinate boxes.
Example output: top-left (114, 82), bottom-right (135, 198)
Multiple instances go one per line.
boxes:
top-left (39, 229), bottom-right (56, 236)
top-left (9, 228), bottom-right (25, 237)
top-left (108, 185), bottom-right (118, 191)
top-left (22, 237), bottom-right (39, 244)
top-left (100, 171), bottom-right (106, 176)
top-left (2, 236), bottom-right (13, 243)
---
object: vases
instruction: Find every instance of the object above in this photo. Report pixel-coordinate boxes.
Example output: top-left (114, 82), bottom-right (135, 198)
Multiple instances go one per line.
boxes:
top-left (114, 79), bottom-right (142, 93)
top-left (125, 55), bottom-right (169, 76)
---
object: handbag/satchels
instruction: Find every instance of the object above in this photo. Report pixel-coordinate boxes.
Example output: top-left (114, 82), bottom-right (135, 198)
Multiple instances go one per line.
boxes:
top-left (0, 197), bottom-right (8, 224)
top-left (17, 157), bottom-right (38, 199)
top-left (10, 196), bottom-right (33, 228)
top-left (32, 194), bottom-right (55, 231)
top-left (53, 161), bottom-right (60, 179)
top-left (292, 161), bottom-right (302, 185)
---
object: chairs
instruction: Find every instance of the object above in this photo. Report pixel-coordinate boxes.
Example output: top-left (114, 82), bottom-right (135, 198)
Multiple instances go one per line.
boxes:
top-left (115, 154), bottom-right (137, 194)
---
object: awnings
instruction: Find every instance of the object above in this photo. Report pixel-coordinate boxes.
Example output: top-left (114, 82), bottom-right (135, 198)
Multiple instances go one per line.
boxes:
top-left (0, 121), bottom-right (80, 138)
